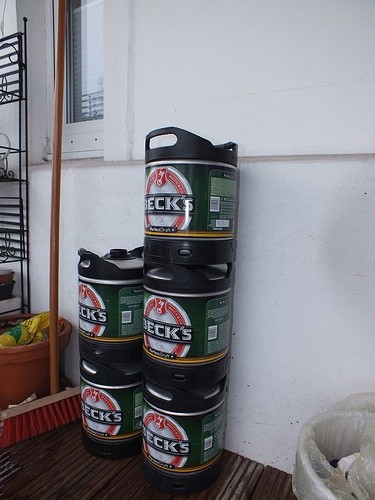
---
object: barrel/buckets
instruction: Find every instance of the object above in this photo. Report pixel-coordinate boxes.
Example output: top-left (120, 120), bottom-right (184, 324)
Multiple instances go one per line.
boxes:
top-left (77, 127), bottom-right (240, 495)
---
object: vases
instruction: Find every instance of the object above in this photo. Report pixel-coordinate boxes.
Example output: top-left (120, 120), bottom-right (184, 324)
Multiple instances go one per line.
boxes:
top-left (0, 312), bottom-right (73, 412)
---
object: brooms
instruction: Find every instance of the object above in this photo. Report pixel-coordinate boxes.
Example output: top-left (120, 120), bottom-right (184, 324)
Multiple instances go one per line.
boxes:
top-left (0, 0), bottom-right (85, 450)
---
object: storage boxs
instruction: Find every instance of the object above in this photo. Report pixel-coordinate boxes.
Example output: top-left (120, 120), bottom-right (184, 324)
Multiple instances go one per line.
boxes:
top-left (0, 267), bottom-right (16, 301)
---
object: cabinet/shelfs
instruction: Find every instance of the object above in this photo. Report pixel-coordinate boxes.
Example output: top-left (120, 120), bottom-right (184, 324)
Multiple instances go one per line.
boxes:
top-left (0, 18), bottom-right (34, 316)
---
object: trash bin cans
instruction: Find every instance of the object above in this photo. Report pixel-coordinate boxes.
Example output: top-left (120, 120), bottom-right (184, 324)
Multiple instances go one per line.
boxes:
top-left (291, 390), bottom-right (375, 500)
